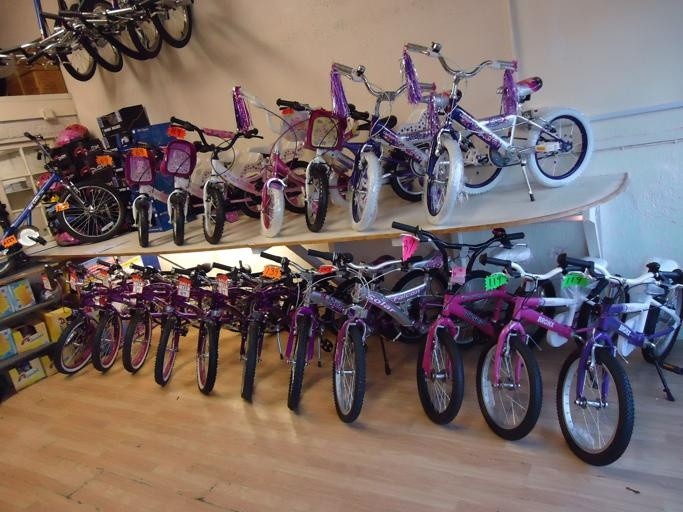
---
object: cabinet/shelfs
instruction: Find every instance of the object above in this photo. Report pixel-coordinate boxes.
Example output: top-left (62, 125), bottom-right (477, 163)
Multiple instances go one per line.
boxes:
top-left (0, 262), bottom-right (62, 395)
top-left (0, 138), bottom-right (69, 252)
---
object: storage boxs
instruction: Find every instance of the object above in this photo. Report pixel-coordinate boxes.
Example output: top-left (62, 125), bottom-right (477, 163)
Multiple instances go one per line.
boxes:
top-left (12, 318), bottom-right (50, 354)
top-left (4, 356), bottom-right (47, 392)
top-left (0, 328), bottom-right (18, 361)
top-left (41, 346), bottom-right (75, 377)
top-left (0, 285), bottom-right (16, 320)
top-left (37, 304), bottom-right (72, 343)
top-left (8, 279), bottom-right (36, 311)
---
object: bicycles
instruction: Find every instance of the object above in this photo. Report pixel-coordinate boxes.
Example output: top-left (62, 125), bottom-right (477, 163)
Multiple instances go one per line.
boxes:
top-left (330, 62), bottom-right (506, 231)
top-left (41, 258), bottom-right (450, 425)
top-left (0, 133), bottom-right (124, 260)
top-left (474, 251), bottom-right (630, 443)
top-left (0, 0), bottom-right (192, 83)
top-left (553, 253), bottom-right (683, 465)
top-left (421, 44), bottom-right (591, 224)
top-left (388, 219), bottom-right (561, 426)
top-left (272, 96), bottom-right (428, 234)
top-left (112, 84), bottom-right (313, 245)
